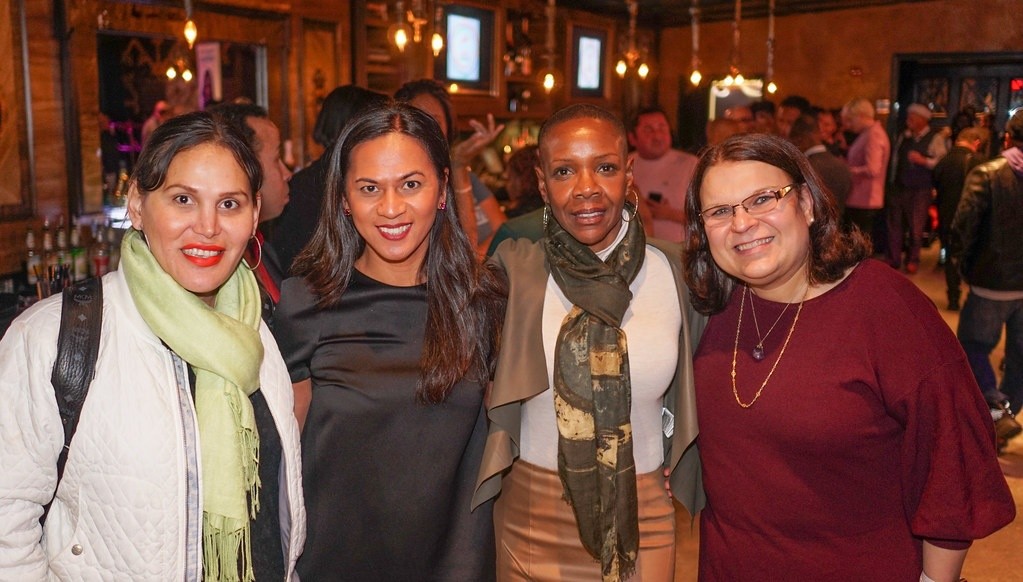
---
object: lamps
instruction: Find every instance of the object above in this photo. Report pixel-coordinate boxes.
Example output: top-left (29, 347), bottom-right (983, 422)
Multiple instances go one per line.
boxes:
top-left (538, 0), bottom-right (565, 95)
top-left (388, 1), bottom-right (413, 55)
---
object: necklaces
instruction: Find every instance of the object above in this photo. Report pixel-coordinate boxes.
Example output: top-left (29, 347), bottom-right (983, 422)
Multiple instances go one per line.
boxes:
top-left (732, 282), bottom-right (811, 407)
top-left (748, 279), bottom-right (806, 360)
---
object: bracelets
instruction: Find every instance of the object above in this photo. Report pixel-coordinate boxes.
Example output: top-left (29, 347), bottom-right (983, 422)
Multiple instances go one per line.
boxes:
top-left (454, 186), bottom-right (472, 193)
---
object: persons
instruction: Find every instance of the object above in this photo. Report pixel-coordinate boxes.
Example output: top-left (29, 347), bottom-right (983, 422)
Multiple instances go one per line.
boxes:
top-left (681, 133), bottom-right (1016, 582)
top-left (273, 104), bottom-right (673, 582)
top-left (469, 103), bottom-right (708, 582)
top-left (226, 78), bottom-right (1007, 312)
top-left (0, 102), bottom-right (307, 582)
top-left (141, 99), bottom-right (171, 144)
top-left (949, 106), bottom-right (1023, 454)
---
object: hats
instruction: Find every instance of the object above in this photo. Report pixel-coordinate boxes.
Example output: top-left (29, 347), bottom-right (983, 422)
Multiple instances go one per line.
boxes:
top-left (907, 103), bottom-right (932, 119)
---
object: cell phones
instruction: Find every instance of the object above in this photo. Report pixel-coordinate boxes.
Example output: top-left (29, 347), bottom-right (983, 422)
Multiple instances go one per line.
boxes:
top-left (648, 191), bottom-right (662, 202)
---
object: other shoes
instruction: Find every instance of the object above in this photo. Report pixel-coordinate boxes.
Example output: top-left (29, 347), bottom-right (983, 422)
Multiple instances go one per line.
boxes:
top-left (989, 401), bottom-right (1022, 454)
top-left (905, 260), bottom-right (919, 275)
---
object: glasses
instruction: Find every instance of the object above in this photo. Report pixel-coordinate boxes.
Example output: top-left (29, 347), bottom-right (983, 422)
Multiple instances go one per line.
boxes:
top-left (698, 183), bottom-right (798, 228)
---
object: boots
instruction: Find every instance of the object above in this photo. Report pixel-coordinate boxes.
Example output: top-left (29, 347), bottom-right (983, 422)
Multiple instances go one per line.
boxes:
top-left (946, 289), bottom-right (961, 311)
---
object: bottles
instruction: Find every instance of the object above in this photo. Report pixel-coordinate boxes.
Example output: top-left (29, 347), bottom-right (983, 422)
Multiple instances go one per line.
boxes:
top-left (14, 215), bottom-right (120, 297)
